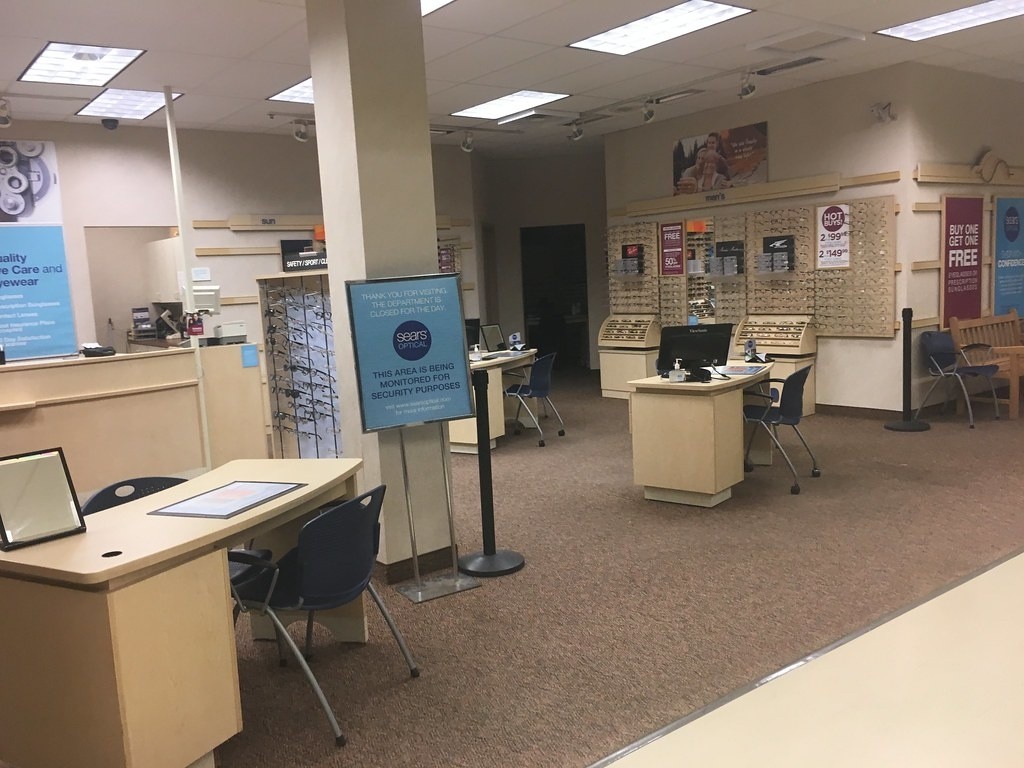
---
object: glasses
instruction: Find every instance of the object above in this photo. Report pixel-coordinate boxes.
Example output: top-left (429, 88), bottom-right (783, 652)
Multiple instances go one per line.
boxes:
top-left (258, 282), bottom-right (340, 442)
top-left (328, 447), bottom-right (345, 456)
top-left (602, 201), bottom-right (888, 347)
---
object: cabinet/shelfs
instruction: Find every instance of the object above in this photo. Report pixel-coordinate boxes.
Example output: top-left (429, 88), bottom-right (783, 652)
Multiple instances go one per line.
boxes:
top-left (598, 349), bottom-right (659, 400)
top-left (730, 356), bottom-right (815, 417)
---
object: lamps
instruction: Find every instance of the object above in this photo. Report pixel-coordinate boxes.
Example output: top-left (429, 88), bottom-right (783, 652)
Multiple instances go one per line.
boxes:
top-left (571, 121), bottom-right (583, 141)
top-left (461, 131), bottom-right (473, 152)
top-left (738, 74), bottom-right (757, 99)
top-left (294, 119), bottom-right (309, 142)
top-left (640, 101), bottom-right (655, 122)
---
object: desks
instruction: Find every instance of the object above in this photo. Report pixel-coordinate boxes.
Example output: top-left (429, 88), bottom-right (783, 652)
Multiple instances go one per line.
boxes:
top-left (0, 459), bottom-right (366, 768)
top-left (448, 349), bottom-right (538, 454)
top-left (626, 362), bottom-right (774, 508)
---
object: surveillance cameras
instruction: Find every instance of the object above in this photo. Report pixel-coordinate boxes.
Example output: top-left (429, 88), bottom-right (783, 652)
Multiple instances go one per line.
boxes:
top-left (102, 119), bottom-right (119, 130)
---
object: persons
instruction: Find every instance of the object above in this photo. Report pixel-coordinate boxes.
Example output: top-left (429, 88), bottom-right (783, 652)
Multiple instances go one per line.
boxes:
top-left (677, 132), bottom-right (735, 194)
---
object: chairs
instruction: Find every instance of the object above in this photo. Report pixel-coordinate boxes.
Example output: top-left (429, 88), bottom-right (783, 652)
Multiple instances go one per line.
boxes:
top-left (80, 477), bottom-right (287, 668)
top-left (502, 352), bottom-right (565, 447)
top-left (743, 364), bottom-right (821, 494)
top-left (914, 331), bottom-right (999, 429)
top-left (229, 484), bottom-right (420, 746)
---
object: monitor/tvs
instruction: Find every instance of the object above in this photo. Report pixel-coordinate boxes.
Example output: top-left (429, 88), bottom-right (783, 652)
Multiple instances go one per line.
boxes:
top-left (657, 324), bottom-right (732, 381)
top-left (465, 319), bottom-right (480, 352)
top-left (192, 286), bottom-right (220, 316)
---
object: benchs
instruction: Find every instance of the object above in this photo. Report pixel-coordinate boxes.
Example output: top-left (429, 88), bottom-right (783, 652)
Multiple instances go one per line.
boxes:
top-left (949, 308), bottom-right (1024, 420)
